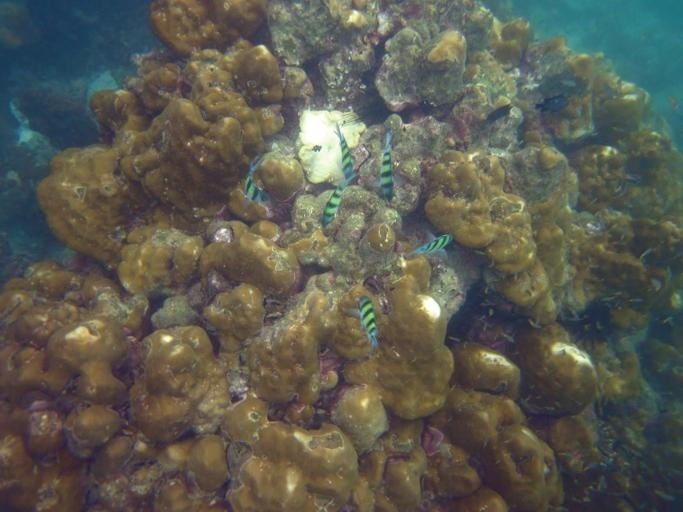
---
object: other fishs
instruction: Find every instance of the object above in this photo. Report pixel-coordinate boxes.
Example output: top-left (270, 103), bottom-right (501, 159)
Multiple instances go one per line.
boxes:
top-left (9, 101), bottom-right (30, 126)
